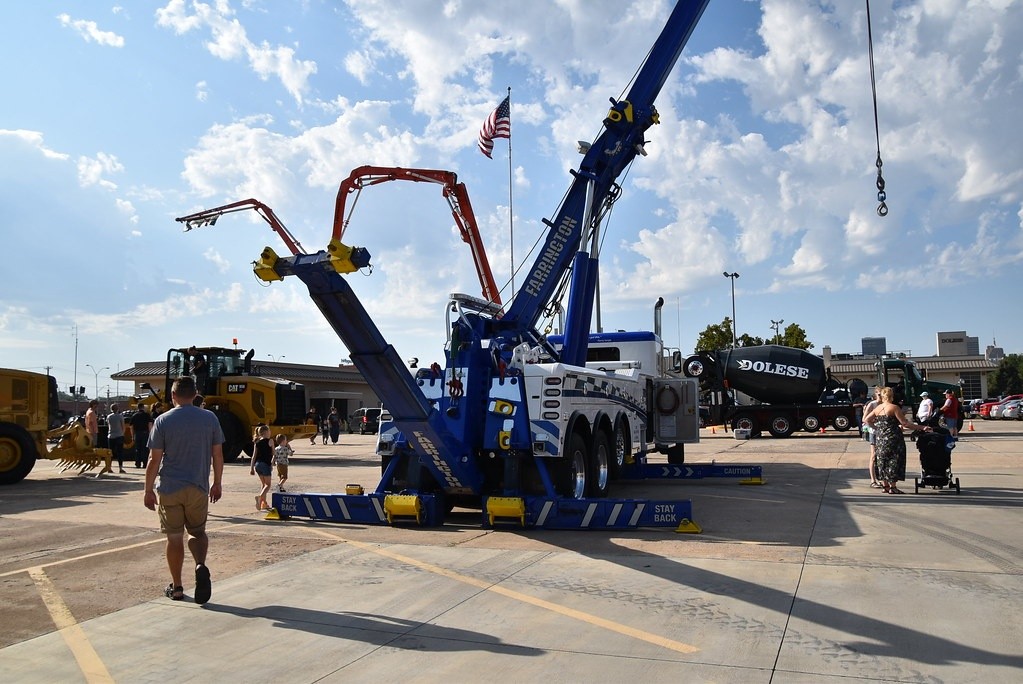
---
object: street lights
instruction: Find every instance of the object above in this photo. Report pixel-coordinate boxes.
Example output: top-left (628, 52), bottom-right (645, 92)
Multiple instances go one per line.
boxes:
top-left (86, 363), bottom-right (109, 403)
top-left (722, 272), bottom-right (739, 348)
top-left (769, 318), bottom-right (783, 344)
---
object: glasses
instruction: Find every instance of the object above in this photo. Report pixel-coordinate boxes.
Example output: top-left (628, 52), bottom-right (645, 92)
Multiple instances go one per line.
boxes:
top-left (877, 393), bottom-right (880, 396)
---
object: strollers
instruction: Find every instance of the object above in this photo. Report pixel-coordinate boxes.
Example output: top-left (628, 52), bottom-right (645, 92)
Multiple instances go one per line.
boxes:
top-left (910, 408), bottom-right (960, 495)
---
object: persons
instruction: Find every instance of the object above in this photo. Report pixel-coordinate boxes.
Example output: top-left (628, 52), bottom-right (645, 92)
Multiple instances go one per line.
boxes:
top-left (916, 388), bottom-right (959, 441)
top-left (271, 434), bottom-right (293, 493)
top-left (862, 376), bottom-right (933, 494)
top-left (853, 390), bottom-right (869, 438)
top-left (192, 394), bottom-right (206, 409)
top-left (185, 346), bottom-right (207, 394)
top-left (144, 376), bottom-right (226, 604)
top-left (304, 406), bottom-right (342, 445)
top-left (85, 399), bottom-right (165, 473)
top-left (250, 425), bottom-right (276, 511)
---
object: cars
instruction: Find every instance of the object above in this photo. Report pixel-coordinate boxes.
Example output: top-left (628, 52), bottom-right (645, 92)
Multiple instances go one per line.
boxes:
top-left (971, 394), bottom-right (1022, 421)
top-left (345, 408), bottom-right (380, 433)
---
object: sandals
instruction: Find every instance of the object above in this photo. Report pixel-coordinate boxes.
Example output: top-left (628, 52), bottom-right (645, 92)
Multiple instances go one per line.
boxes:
top-left (881, 485), bottom-right (890, 493)
top-left (163, 583), bottom-right (184, 600)
top-left (870, 482), bottom-right (884, 488)
top-left (889, 486), bottom-right (905, 494)
top-left (194, 564), bottom-right (212, 605)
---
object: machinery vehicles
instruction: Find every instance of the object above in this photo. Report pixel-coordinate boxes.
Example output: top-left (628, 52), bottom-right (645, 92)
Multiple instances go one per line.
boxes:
top-left (683, 344), bottom-right (963, 445)
top-left (378, 0), bottom-right (892, 511)
top-left (0, 366), bottom-right (113, 486)
top-left (54, 348), bottom-right (319, 480)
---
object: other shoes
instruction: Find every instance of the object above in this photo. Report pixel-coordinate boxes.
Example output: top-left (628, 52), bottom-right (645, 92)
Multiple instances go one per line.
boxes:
top-left (119, 470), bottom-right (126, 473)
top-left (108, 470), bottom-right (113, 473)
top-left (332, 442), bottom-right (335, 445)
top-left (275, 484), bottom-right (281, 493)
top-left (280, 489), bottom-right (286, 492)
top-left (954, 437), bottom-right (958, 441)
top-left (254, 495), bottom-right (262, 511)
top-left (261, 504), bottom-right (270, 510)
top-left (311, 440), bottom-right (315, 445)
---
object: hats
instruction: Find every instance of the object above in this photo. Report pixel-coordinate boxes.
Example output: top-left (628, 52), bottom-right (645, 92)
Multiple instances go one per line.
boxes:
top-left (942, 389), bottom-right (953, 394)
top-left (920, 392), bottom-right (929, 396)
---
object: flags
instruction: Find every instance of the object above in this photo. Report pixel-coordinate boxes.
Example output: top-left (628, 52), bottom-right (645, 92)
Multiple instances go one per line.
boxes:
top-left (477, 94), bottom-right (511, 159)
